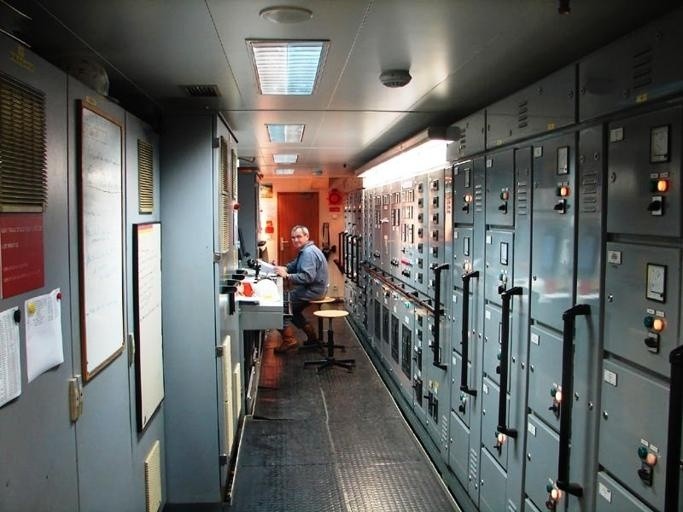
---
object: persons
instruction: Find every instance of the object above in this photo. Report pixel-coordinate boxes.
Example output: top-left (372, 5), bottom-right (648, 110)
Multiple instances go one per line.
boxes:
top-left (273, 224), bottom-right (330, 353)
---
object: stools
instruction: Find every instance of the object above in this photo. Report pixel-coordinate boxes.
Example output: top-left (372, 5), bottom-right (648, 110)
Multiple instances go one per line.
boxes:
top-left (299, 296), bottom-right (356, 376)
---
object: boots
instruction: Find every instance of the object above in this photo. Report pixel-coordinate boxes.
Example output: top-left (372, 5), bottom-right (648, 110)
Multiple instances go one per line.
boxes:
top-left (274, 325), bottom-right (299, 355)
top-left (302, 322), bottom-right (317, 345)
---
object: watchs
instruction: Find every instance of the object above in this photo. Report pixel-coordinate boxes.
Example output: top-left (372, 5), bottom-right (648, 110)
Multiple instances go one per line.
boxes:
top-left (286, 273), bottom-right (290, 280)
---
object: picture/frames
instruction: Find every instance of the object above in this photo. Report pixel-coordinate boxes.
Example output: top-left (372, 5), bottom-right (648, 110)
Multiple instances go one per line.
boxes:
top-left (75, 98), bottom-right (168, 435)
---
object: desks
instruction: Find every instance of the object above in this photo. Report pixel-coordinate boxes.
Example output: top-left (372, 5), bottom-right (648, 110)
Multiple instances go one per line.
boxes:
top-left (239, 266), bottom-right (284, 333)
